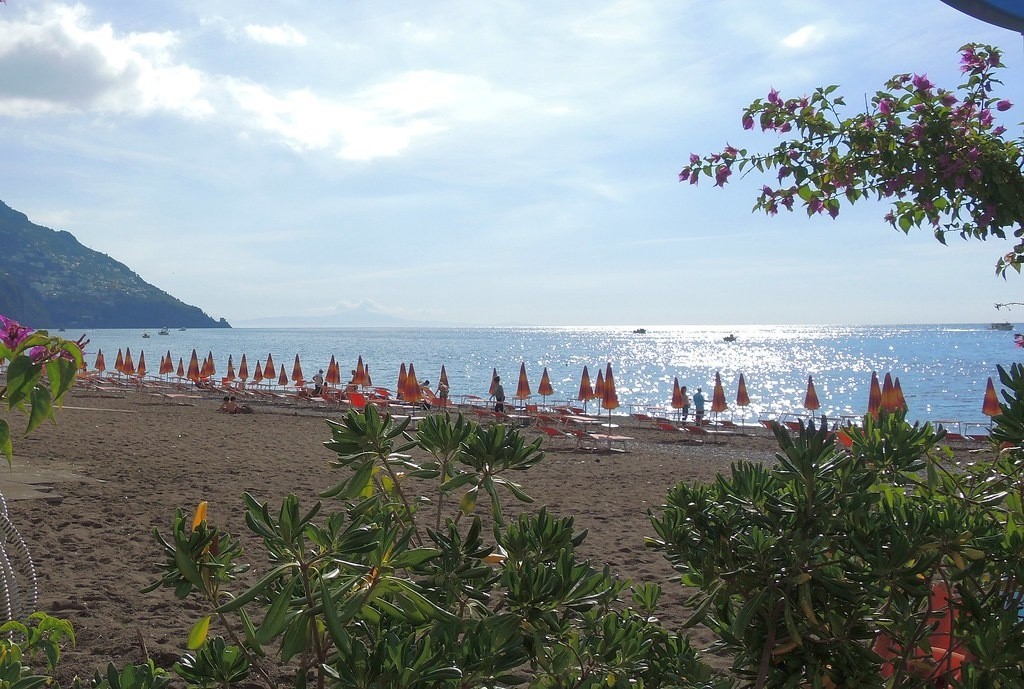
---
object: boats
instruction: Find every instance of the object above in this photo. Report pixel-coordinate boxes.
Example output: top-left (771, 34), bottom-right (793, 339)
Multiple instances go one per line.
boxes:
top-left (723, 336), bottom-right (737, 342)
top-left (988, 322), bottom-right (1013, 331)
top-left (634, 328), bottom-right (647, 333)
top-left (179, 327), bottom-right (186, 331)
top-left (158, 326), bottom-right (170, 335)
top-left (143, 333), bottom-right (150, 338)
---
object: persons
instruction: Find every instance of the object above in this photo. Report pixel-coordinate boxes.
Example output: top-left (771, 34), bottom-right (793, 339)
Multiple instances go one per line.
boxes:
top-left (84, 362), bottom-right (87, 372)
top-left (216, 396), bottom-right (249, 414)
top-left (420, 381), bottom-right (429, 394)
top-left (312, 369), bottom-right (324, 394)
top-left (342, 370), bottom-right (358, 393)
top-left (434, 382), bottom-right (449, 412)
top-left (490, 377), bottom-right (505, 413)
top-left (681, 386), bottom-right (704, 426)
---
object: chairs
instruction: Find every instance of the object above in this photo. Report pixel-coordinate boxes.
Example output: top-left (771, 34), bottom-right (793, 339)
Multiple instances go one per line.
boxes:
top-left (963, 422), bottom-right (997, 449)
top-left (72, 370), bottom-right (871, 459)
top-left (933, 420), bottom-right (968, 451)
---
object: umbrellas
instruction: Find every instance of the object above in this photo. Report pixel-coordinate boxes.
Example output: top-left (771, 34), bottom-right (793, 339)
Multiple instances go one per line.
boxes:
top-left (517, 362), bottom-right (531, 414)
top-left (489, 368), bottom-right (498, 407)
top-left (982, 377), bottom-right (1002, 435)
top-left (397, 363), bottom-right (421, 416)
top-left (804, 376), bottom-right (820, 423)
top-left (439, 365), bottom-right (450, 388)
top-left (671, 378), bottom-right (684, 423)
top-left (578, 362), bottom-right (620, 450)
top-left (736, 374), bottom-right (750, 426)
top-left (868, 372), bottom-right (907, 419)
top-left (538, 367), bottom-right (553, 404)
top-left (711, 373), bottom-right (726, 431)
top-left (95, 348), bottom-right (373, 398)
